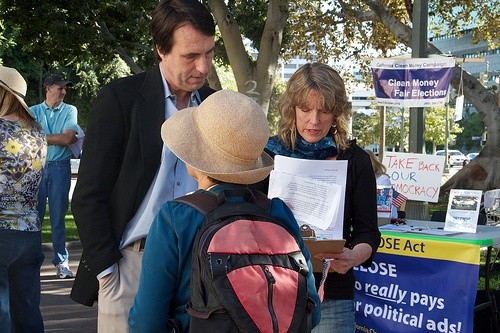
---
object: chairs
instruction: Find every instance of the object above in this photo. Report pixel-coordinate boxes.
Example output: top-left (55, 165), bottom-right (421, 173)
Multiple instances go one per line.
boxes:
top-left (432, 211), bottom-right (487, 225)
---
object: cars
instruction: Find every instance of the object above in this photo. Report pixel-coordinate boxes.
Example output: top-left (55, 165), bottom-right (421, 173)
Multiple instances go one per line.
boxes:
top-left (435, 150), bottom-right (467, 167)
top-left (468, 152), bottom-right (480, 161)
top-left (70, 148), bottom-right (82, 174)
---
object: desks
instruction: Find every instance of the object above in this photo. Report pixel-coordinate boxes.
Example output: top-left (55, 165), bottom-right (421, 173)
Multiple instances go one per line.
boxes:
top-left (353, 224), bottom-right (500, 333)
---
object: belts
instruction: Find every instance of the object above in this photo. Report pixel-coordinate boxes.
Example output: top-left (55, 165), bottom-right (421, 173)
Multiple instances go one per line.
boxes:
top-left (130, 237), bottom-right (146, 251)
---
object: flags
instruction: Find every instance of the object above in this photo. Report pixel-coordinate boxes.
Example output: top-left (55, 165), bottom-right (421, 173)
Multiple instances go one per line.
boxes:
top-left (392, 188), bottom-right (408, 209)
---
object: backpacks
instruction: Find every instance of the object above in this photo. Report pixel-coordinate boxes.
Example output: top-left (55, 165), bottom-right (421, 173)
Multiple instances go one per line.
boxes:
top-left (172, 191), bottom-right (314, 333)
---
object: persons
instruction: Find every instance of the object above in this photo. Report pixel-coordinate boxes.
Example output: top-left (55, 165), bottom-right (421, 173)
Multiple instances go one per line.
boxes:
top-left (264, 63), bottom-right (381, 333)
top-left (28, 73), bottom-right (78, 279)
top-left (0, 66), bottom-right (48, 332)
top-left (71, 1), bottom-right (218, 333)
top-left (126, 90), bottom-right (321, 332)
top-left (364, 150), bottom-right (392, 221)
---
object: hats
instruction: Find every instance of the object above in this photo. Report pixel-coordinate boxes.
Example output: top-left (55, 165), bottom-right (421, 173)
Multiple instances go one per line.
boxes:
top-left (0, 66), bottom-right (36, 119)
top-left (44, 74), bottom-right (72, 87)
top-left (161, 90), bottom-right (274, 184)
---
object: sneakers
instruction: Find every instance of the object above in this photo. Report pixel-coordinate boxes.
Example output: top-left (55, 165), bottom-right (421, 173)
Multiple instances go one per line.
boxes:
top-left (56, 262), bottom-right (73, 279)
